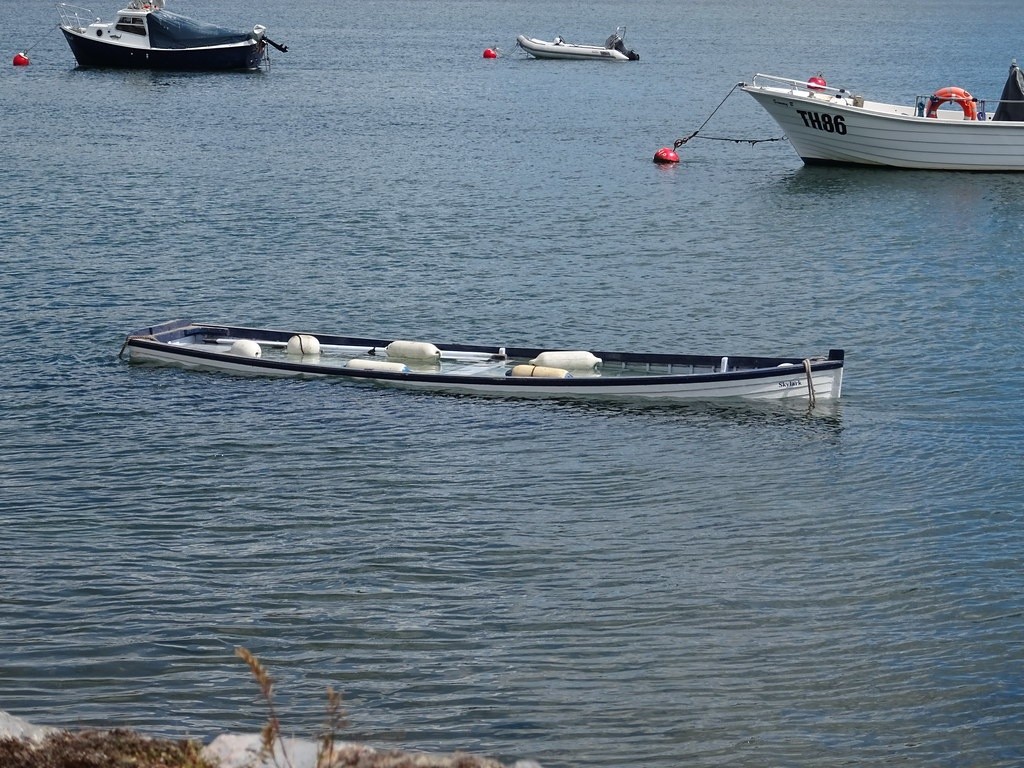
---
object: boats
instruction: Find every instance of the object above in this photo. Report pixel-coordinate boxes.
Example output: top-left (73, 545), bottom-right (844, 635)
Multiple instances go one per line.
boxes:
top-left (738, 69), bottom-right (1023, 173)
top-left (49, 1), bottom-right (292, 72)
top-left (514, 25), bottom-right (641, 63)
top-left (114, 308), bottom-right (851, 412)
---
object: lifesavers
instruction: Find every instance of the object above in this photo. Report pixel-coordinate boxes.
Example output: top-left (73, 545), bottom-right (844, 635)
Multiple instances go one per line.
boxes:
top-left (925, 86), bottom-right (977, 121)
